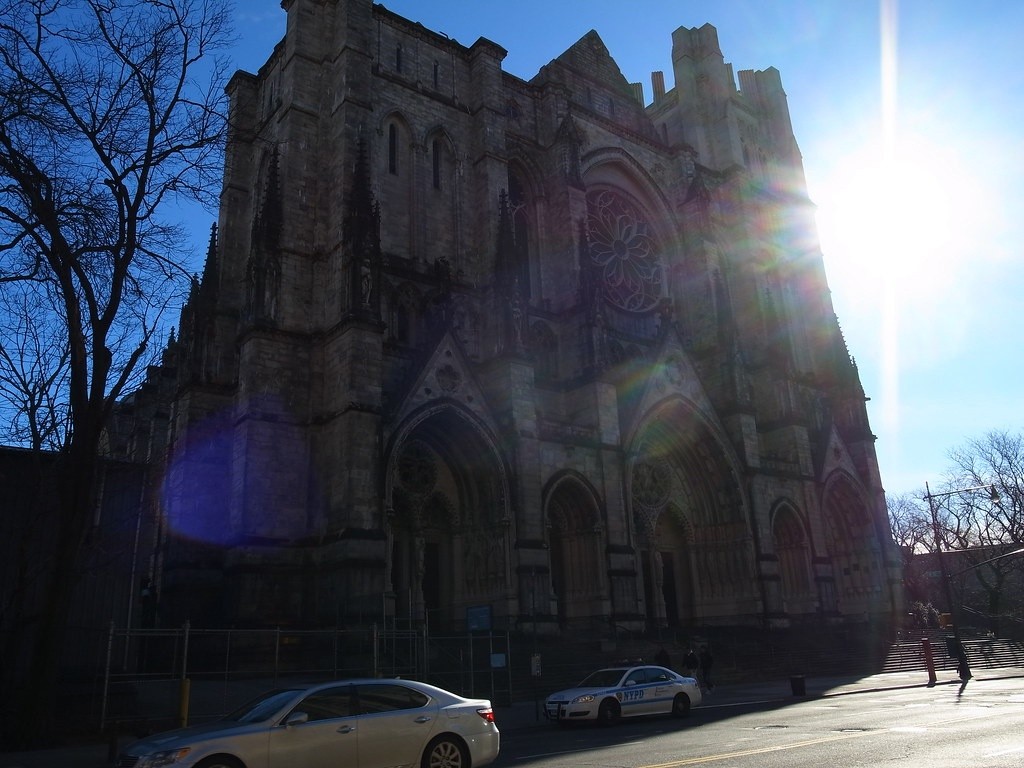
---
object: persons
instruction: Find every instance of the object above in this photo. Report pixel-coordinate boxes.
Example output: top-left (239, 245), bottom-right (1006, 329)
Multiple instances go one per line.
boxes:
top-left (682, 647), bottom-right (699, 682)
top-left (698, 645), bottom-right (716, 695)
top-left (653, 646), bottom-right (673, 668)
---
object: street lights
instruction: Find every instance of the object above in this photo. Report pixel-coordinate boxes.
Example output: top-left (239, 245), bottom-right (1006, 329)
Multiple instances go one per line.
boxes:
top-left (924, 478), bottom-right (1001, 679)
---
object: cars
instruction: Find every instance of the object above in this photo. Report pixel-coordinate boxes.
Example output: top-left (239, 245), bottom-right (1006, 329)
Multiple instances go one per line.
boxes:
top-left (116, 673), bottom-right (500, 768)
top-left (545, 658), bottom-right (703, 721)
